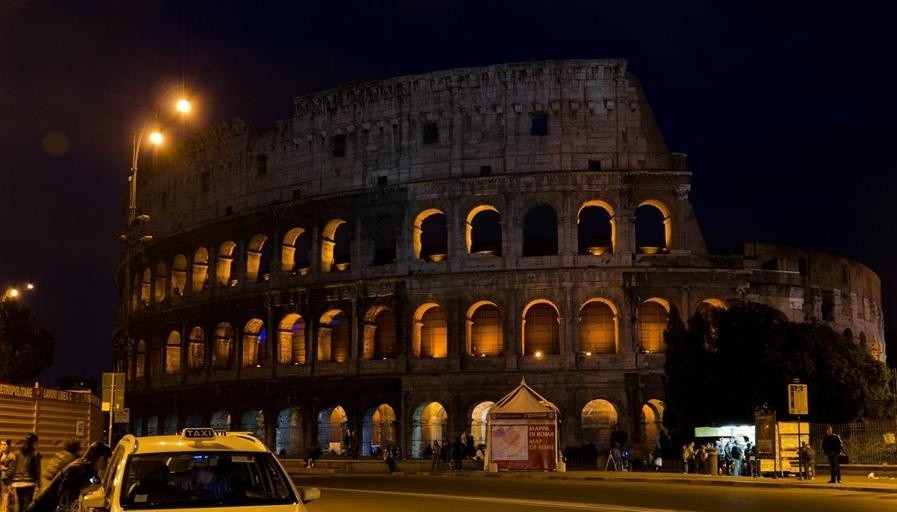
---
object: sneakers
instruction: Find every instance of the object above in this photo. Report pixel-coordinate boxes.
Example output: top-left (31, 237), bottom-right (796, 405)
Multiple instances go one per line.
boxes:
top-left (828, 479), bottom-right (842, 483)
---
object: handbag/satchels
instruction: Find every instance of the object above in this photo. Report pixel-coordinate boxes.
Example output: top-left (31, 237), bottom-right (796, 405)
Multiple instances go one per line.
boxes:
top-left (839, 454), bottom-right (850, 464)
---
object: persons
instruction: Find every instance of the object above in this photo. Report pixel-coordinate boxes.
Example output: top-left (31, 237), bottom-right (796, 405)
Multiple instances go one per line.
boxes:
top-left (822, 425), bottom-right (843, 483)
top-left (796, 441), bottom-right (815, 480)
top-left (11, 433), bottom-right (41, 511)
top-left (565, 439), bottom-right (758, 476)
top-left (0, 437), bottom-right (16, 512)
top-left (39, 438), bottom-right (83, 493)
top-left (276, 429), bottom-right (484, 475)
top-left (25, 440), bottom-right (113, 512)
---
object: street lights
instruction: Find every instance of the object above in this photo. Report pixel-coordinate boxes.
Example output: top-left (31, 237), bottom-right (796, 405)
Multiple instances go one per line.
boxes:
top-left (123, 79), bottom-right (200, 434)
top-left (6, 283), bottom-right (36, 383)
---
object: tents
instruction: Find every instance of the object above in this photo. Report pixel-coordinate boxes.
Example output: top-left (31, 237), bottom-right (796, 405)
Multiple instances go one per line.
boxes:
top-left (483, 376), bottom-right (562, 472)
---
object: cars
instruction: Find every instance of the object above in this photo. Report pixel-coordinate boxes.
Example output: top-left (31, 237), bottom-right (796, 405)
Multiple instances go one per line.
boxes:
top-left (79, 426), bottom-right (321, 512)
